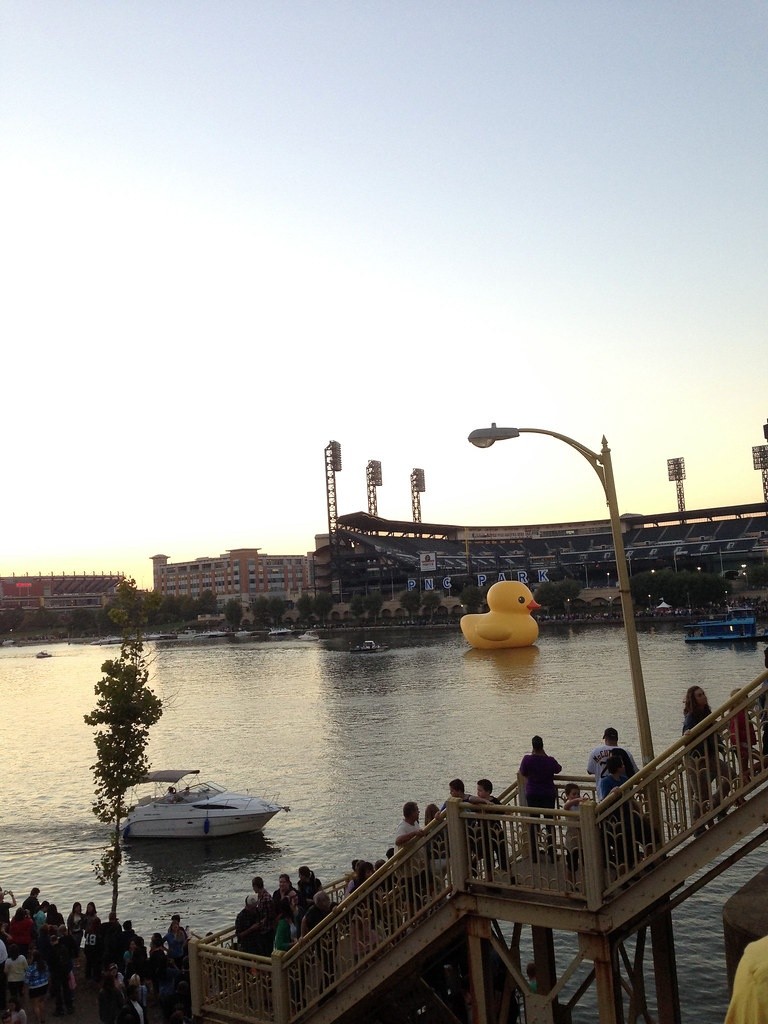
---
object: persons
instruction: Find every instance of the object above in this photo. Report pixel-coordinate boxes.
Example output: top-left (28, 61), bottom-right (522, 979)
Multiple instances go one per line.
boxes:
top-left (234, 895), bottom-right (273, 1012)
top-left (759, 646), bottom-right (768, 768)
top-left (469, 779), bottom-right (509, 873)
top-left (386, 848), bottom-right (394, 858)
top-left (681, 685), bottom-right (736, 834)
top-left (520, 736), bottom-right (562, 864)
top-left (299, 890), bottom-right (341, 994)
top-left (563, 783), bottom-right (590, 893)
top-left (99, 586), bottom-right (768, 660)
top-left (597, 756), bottom-right (661, 871)
top-left (0, 886), bottom-right (221, 1024)
top-left (283, 888), bottom-right (303, 931)
top-left (251, 877), bottom-right (273, 935)
top-left (608, 748), bottom-right (636, 780)
top-left (272, 873), bottom-right (298, 922)
top-left (344, 862), bottom-right (385, 956)
top-left (395, 802), bottom-right (429, 920)
top-left (273, 895), bottom-right (301, 952)
top-left (375, 859), bottom-right (396, 936)
top-left (424, 804), bottom-right (450, 876)
top-left (729, 688), bottom-right (757, 783)
top-left (433, 778), bottom-right (494, 821)
top-left (586, 727), bottom-right (640, 803)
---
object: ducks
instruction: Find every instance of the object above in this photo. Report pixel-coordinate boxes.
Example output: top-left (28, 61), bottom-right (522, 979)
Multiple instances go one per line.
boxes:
top-left (460, 581), bottom-right (542, 649)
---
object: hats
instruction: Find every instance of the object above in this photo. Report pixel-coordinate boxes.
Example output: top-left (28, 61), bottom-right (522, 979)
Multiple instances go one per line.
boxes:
top-left (602, 727), bottom-right (618, 740)
top-left (246, 895), bottom-right (258, 906)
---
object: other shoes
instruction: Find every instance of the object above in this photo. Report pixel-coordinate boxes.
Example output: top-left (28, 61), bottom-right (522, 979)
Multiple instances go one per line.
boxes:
top-left (614, 878), bottom-right (631, 889)
top-left (510, 876), bottom-right (520, 885)
top-left (532, 855), bottom-right (559, 864)
top-left (53, 1007), bottom-right (76, 1016)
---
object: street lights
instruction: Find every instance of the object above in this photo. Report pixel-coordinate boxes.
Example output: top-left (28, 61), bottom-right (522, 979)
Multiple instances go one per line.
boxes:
top-left (469, 421), bottom-right (662, 836)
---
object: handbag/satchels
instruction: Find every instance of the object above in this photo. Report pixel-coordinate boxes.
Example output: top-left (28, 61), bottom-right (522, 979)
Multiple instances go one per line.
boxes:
top-left (79, 929), bottom-right (87, 948)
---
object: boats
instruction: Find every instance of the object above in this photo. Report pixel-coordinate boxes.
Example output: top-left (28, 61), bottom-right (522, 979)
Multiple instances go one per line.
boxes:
top-left (298, 631), bottom-right (320, 641)
top-left (119, 770), bottom-right (290, 837)
top-left (2, 639), bottom-right (14, 645)
top-left (37, 652), bottom-right (52, 658)
top-left (90, 629), bottom-right (252, 645)
top-left (268, 628), bottom-right (293, 635)
top-left (349, 640), bottom-right (388, 653)
top-left (684, 607), bottom-right (768, 642)
